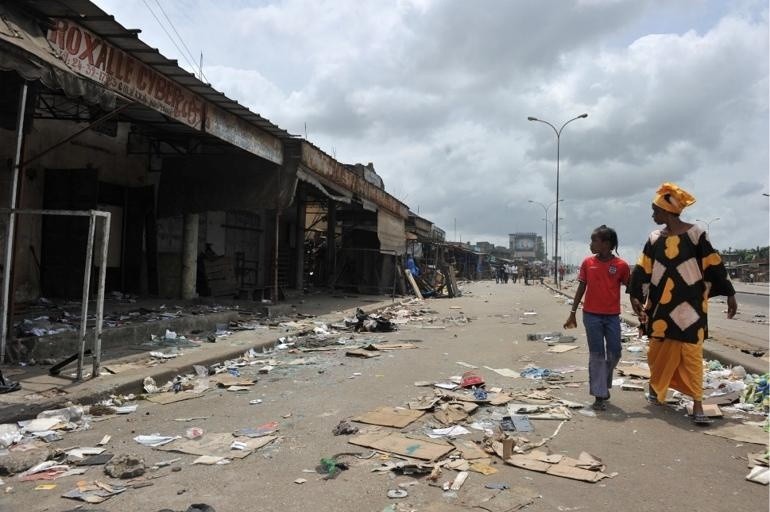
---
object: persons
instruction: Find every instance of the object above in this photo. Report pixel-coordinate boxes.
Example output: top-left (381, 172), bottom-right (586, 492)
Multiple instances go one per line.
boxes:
top-left (563, 224), bottom-right (651, 410)
top-left (626, 182), bottom-right (738, 424)
top-left (492, 262), bottom-right (569, 285)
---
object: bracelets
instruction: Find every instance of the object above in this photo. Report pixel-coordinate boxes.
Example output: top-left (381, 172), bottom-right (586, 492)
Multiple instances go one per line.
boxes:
top-left (569, 310), bottom-right (576, 313)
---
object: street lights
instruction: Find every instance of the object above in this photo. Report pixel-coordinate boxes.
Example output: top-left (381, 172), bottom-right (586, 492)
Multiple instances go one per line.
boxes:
top-left (527, 114), bottom-right (588, 282)
top-left (696, 218), bottom-right (720, 237)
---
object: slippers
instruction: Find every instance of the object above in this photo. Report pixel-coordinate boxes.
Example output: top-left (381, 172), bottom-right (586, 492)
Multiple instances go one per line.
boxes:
top-left (693, 413), bottom-right (713, 423)
top-left (645, 392), bottom-right (664, 406)
top-left (593, 389), bottom-right (610, 410)
